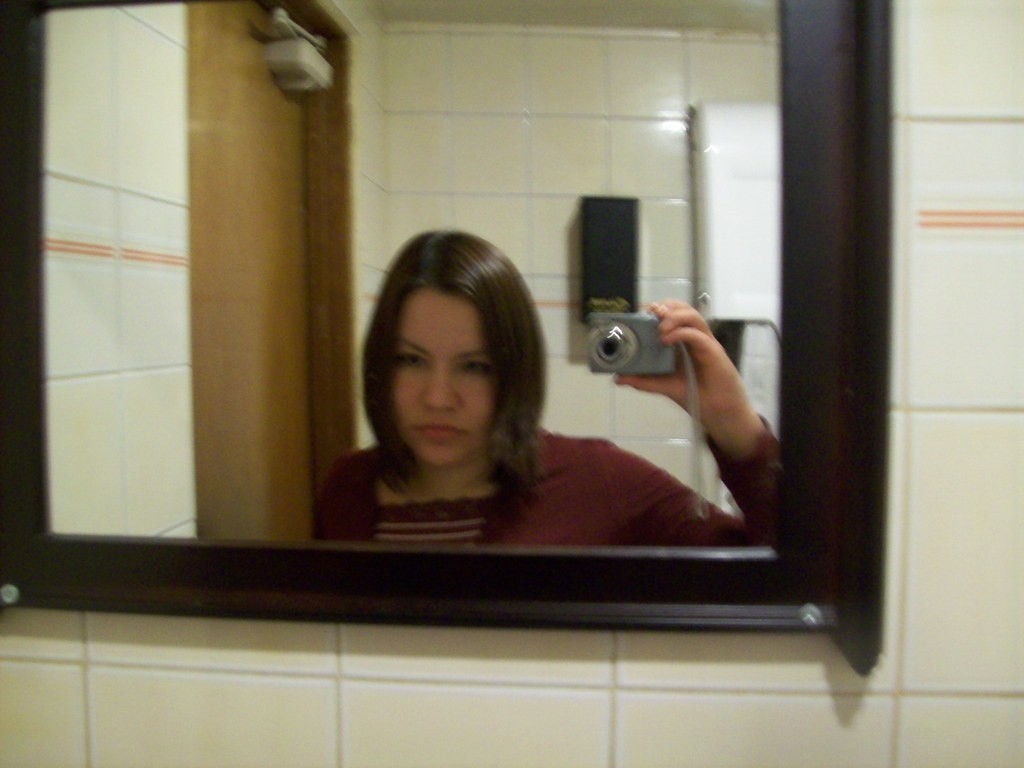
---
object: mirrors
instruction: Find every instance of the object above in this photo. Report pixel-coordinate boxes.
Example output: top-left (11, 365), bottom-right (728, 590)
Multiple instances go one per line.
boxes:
top-left (0, 1), bottom-right (897, 678)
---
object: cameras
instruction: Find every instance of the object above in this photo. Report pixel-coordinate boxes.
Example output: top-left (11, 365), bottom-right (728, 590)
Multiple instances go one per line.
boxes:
top-left (588, 311), bottom-right (678, 375)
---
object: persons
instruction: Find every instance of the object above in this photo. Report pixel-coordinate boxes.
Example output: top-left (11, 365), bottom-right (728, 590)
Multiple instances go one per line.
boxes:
top-left (307, 229), bottom-right (779, 547)
